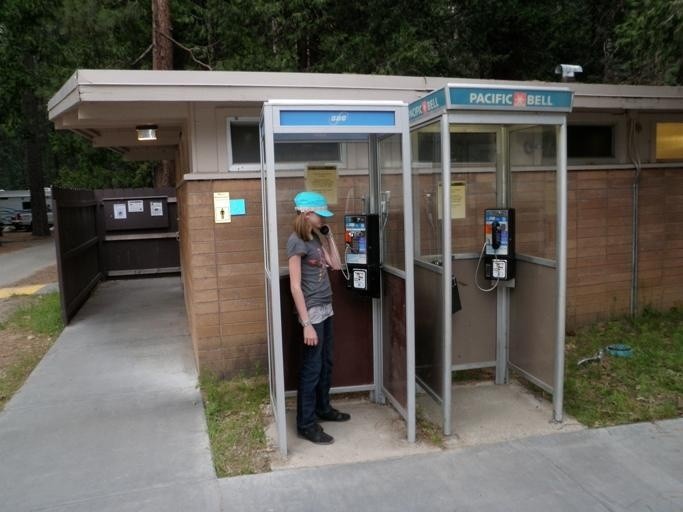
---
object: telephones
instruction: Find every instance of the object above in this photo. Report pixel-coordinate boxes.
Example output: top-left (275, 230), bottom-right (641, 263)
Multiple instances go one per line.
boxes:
top-left (492, 221), bottom-right (501, 249)
top-left (320, 225), bottom-right (329, 235)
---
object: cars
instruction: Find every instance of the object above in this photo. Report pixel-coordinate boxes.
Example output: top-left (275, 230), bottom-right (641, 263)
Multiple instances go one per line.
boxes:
top-left (11, 208), bottom-right (53, 232)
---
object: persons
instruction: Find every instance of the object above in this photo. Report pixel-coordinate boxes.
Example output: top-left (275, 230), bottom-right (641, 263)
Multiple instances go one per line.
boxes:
top-left (285, 191), bottom-right (352, 444)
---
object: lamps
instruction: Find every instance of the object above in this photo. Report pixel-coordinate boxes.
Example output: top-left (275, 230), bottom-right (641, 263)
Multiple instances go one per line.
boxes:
top-left (137, 123), bottom-right (159, 142)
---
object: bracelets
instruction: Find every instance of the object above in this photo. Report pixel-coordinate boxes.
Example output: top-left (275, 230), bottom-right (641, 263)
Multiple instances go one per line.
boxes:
top-left (300, 320), bottom-right (311, 327)
top-left (325, 233), bottom-right (333, 240)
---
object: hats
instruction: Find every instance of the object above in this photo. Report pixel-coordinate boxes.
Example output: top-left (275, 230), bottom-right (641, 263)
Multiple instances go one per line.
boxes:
top-left (293, 190), bottom-right (334, 218)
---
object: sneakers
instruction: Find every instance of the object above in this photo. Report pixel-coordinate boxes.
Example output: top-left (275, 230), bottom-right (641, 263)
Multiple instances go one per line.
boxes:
top-left (314, 404), bottom-right (351, 422)
top-left (297, 421), bottom-right (335, 445)
